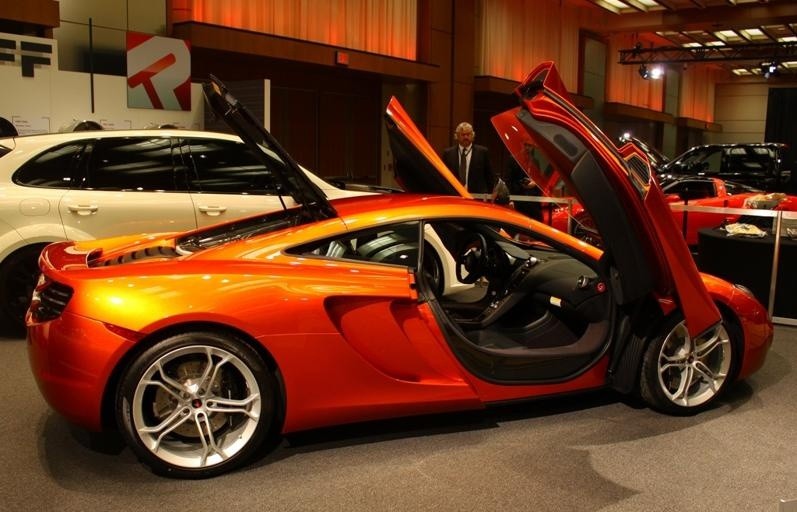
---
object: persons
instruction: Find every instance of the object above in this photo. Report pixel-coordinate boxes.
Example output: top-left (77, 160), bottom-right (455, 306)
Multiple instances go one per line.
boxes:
top-left (504, 142), bottom-right (541, 222)
top-left (438, 122), bottom-right (494, 199)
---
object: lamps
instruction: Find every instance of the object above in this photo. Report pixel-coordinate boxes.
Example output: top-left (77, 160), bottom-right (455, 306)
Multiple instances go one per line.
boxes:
top-left (758, 56), bottom-right (781, 81)
top-left (638, 63), bottom-right (654, 81)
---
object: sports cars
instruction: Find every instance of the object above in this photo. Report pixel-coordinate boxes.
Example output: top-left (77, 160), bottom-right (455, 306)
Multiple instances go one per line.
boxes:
top-left (34, 63), bottom-right (776, 485)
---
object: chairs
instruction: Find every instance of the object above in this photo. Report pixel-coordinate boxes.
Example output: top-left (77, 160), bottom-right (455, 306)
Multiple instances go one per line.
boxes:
top-left (422, 249), bottom-right (528, 349)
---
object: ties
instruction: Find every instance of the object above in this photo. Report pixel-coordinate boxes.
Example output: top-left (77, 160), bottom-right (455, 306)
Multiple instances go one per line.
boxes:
top-left (459, 147), bottom-right (467, 186)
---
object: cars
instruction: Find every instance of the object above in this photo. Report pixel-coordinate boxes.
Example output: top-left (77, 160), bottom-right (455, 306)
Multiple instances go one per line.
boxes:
top-left (518, 130), bottom-right (797, 264)
top-left (1, 127), bottom-right (480, 345)
